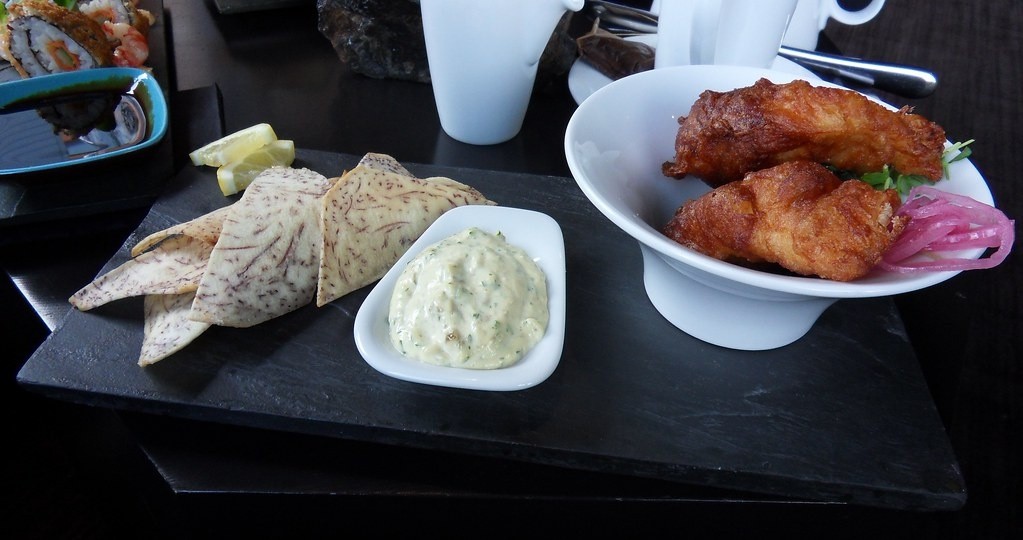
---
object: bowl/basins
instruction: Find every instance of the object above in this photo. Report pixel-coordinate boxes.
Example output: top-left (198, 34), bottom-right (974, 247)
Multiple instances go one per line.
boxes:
top-left (564, 63), bottom-right (997, 352)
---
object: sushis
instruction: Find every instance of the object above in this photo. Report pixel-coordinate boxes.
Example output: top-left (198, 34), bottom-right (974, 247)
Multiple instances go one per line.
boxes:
top-left (0, 0), bottom-right (151, 81)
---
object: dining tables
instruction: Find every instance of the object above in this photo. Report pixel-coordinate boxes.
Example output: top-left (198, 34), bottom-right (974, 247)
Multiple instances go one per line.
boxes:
top-left (0, 0), bottom-right (1023, 540)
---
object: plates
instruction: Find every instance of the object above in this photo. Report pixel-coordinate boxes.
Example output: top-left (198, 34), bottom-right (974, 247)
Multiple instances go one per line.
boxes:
top-left (352, 203), bottom-right (567, 392)
top-left (567, 33), bottom-right (822, 107)
top-left (0, 67), bottom-right (168, 176)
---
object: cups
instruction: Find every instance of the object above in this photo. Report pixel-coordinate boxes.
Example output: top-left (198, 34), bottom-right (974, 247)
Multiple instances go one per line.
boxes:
top-left (780, 0), bottom-right (886, 52)
top-left (420, 0), bottom-right (582, 146)
top-left (653, 0), bottom-right (800, 72)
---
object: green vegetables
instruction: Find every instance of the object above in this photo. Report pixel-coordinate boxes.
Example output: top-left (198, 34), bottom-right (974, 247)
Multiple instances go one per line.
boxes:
top-left (823, 138), bottom-right (976, 196)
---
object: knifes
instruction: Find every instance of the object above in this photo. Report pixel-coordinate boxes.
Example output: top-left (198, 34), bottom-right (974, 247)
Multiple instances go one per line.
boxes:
top-left (585, 0), bottom-right (938, 100)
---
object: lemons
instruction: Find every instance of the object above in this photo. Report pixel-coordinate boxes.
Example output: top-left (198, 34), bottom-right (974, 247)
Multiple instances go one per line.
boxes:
top-left (189, 123), bottom-right (294, 195)
